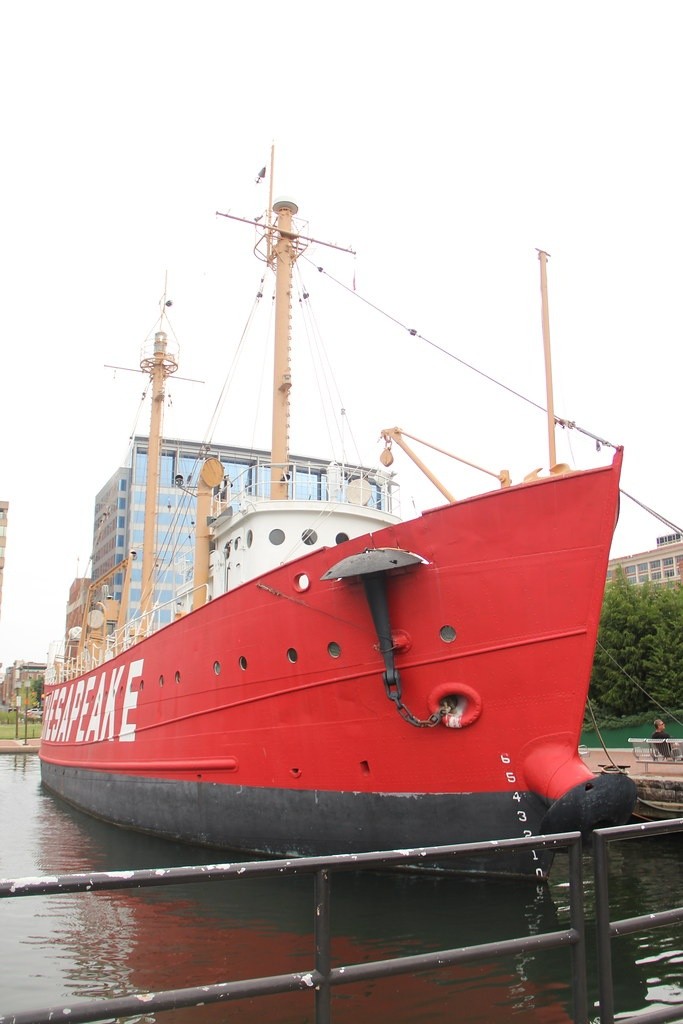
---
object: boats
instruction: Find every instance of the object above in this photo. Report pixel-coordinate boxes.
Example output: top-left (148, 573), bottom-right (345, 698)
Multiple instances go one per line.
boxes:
top-left (38, 140), bottom-right (624, 884)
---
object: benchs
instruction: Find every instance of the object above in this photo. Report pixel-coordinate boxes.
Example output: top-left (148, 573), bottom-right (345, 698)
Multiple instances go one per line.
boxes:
top-left (627, 737), bottom-right (683, 774)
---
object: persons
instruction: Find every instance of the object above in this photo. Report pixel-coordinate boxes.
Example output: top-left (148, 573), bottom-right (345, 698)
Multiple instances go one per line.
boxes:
top-left (652, 719), bottom-right (683, 761)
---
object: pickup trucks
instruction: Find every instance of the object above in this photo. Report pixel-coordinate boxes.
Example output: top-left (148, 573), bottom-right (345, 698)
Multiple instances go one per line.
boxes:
top-left (30, 709), bottom-right (43, 716)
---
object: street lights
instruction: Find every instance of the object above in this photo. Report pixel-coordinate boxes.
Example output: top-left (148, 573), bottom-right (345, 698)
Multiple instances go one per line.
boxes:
top-left (15, 681), bottom-right (21, 738)
top-left (23, 680), bottom-right (30, 746)
